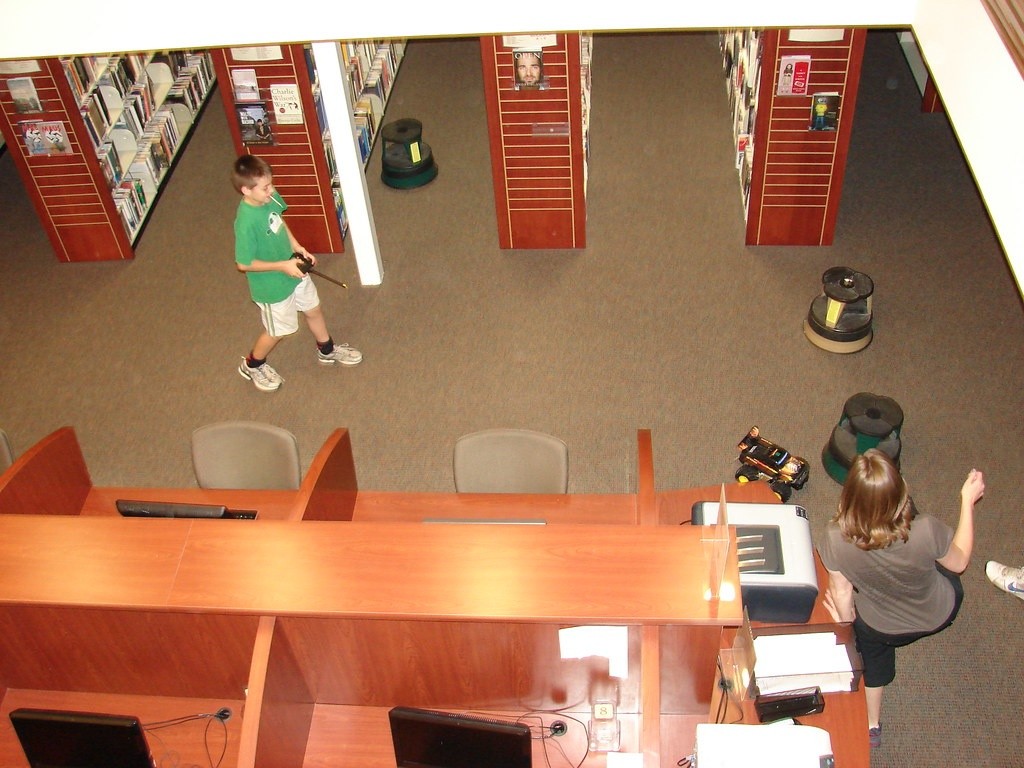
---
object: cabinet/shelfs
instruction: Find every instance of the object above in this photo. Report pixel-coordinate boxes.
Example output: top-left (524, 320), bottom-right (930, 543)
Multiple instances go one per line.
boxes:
top-left (721, 27), bottom-right (867, 248)
top-left (0, 45), bottom-right (216, 262)
top-left (480, 31), bottom-right (595, 249)
top-left (208, 37), bottom-right (406, 254)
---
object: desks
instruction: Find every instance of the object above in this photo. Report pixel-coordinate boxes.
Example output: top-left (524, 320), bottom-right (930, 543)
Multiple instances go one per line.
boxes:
top-left (0, 428), bottom-right (875, 768)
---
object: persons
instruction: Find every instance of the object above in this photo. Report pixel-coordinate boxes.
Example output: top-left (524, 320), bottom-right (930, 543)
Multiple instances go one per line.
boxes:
top-left (22, 124), bottom-right (42, 150)
top-left (985, 561), bottom-right (1024, 601)
top-left (231, 154), bottom-right (363, 391)
top-left (293, 102), bottom-right (298, 109)
top-left (255, 119), bottom-right (271, 142)
top-left (821, 448), bottom-right (985, 748)
top-left (41, 124), bottom-right (66, 150)
top-left (783, 64), bottom-right (792, 84)
top-left (815, 98), bottom-right (827, 132)
top-left (516, 52), bottom-right (543, 84)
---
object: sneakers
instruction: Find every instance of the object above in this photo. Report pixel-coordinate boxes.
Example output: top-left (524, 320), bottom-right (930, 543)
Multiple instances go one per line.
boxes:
top-left (237, 355), bottom-right (282, 391)
top-left (985, 560), bottom-right (1024, 600)
top-left (316, 342), bottom-right (363, 364)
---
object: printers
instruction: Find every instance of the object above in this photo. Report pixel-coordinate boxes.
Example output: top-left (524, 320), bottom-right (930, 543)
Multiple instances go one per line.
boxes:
top-left (692, 499), bottom-right (818, 623)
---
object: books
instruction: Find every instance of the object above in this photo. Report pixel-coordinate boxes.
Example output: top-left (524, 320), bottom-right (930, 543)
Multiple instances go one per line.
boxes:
top-left (235, 102), bottom-right (274, 146)
top-left (232, 69), bottom-right (261, 103)
top-left (303, 35), bottom-right (408, 241)
top-left (580, 32), bottom-right (593, 198)
top-left (718, 27), bottom-right (765, 220)
top-left (270, 84), bottom-right (305, 124)
top-left (17, 120), bottom-right (43, 155)
top-left (6, 77), bottom-right (43, 114)
top-left (58, 49), bottom-right (214, 243)
top-left (512, 48), bottom-right (545, 91)
top-left (36, 121), bottom-right (73, 153)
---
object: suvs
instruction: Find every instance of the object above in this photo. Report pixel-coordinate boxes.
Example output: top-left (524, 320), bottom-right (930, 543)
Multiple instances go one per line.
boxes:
top-left (734, 422), bottom-right (810, 506)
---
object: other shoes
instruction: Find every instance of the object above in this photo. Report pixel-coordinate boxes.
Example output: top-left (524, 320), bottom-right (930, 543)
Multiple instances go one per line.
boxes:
top-left (869, 723), bottom-right (882, 747)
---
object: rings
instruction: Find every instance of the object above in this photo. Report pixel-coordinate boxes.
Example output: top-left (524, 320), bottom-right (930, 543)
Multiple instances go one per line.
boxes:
top-left (830, 602), bottom-right (833, 606)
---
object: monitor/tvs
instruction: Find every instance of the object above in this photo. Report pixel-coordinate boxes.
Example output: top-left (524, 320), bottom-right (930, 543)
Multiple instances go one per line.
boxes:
top-left (388, 706), bottom-right (533, 768)
top-left (9, 706), bottom-right (157, 768)
top-left (116, 499), bottom-right (236, 519)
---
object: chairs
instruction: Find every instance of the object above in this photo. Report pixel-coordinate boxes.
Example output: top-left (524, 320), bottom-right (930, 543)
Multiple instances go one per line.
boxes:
top-left (189, 421), bottom-right (302, 490)
top-left (452, 428), bottom-right (568, 494)
